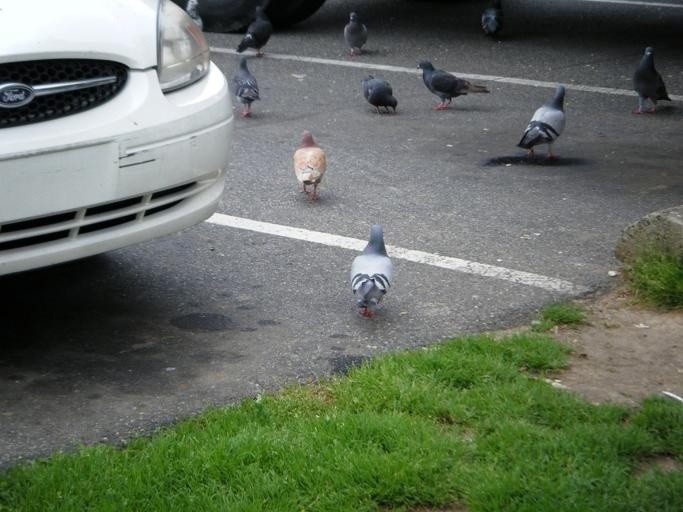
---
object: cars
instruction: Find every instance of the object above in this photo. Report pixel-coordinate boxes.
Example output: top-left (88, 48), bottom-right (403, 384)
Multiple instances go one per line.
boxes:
top-left (0, 0), bottom-right (235, 277)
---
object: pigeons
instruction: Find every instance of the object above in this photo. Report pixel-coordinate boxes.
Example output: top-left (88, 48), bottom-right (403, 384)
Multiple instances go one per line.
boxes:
top-left (231, 57), bottom-right (261, 117)
top-left (349, 224), bottom-right (393, 318)
top-left (416, 60), bottom-right (491, 110)
top-left (293, 129), bottom-right (326, 200)
top-left (479, 0), bottom-right (504, 43)
top-left (514, 85), bottom-right (566, 163)
top-left (360, 74), bottom-right (398, 114)
top-left (186, 0), bottom-right (203, 33)
top-left (344, 12), bottom-right (368, 58)
top-left (236, 6), bottom-right (271, 57)
top-left (631, 47), bottom-right (672, 114)
